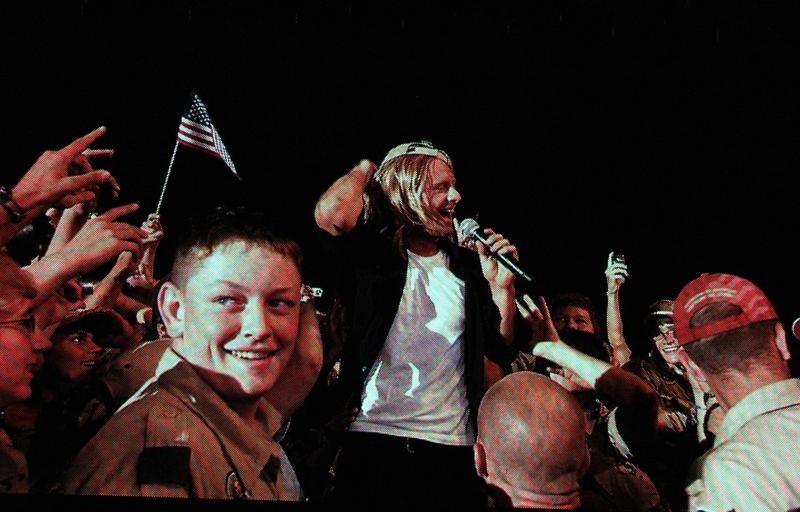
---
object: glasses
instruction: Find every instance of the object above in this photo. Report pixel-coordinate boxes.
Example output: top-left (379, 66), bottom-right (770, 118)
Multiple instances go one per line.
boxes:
top-left (0, 315), bottom-right (35, 333)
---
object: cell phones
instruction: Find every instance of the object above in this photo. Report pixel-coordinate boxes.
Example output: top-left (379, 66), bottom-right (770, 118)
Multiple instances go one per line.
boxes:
top-left (612, 254), bottom-right (625, 276)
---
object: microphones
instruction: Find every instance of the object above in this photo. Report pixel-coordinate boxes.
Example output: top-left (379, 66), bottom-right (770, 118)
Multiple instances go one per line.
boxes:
top-left (460, 218), bottom-right (532, 281)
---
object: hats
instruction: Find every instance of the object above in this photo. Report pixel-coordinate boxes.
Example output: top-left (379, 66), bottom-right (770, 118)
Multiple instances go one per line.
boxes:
top-left (57, 306), bottom-right (134, 349)
top-left (373, 140), bottom-right (453, 179)
top-left (649, 272), bottom-right (779, 346)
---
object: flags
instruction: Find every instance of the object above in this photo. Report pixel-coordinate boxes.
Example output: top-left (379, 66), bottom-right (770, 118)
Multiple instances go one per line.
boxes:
top-left (177, 92), bottom-right (239, 174)
